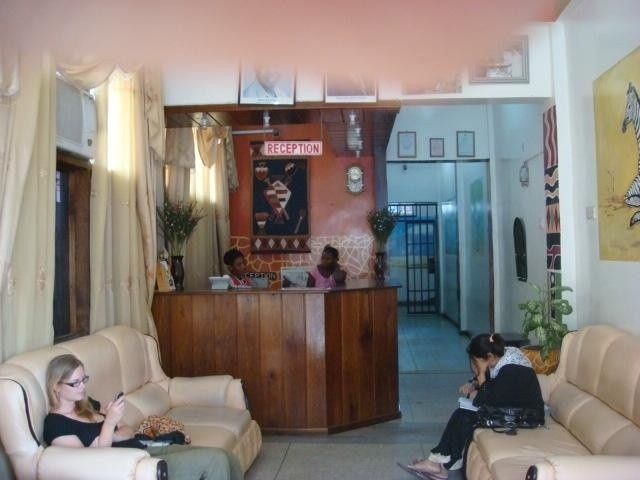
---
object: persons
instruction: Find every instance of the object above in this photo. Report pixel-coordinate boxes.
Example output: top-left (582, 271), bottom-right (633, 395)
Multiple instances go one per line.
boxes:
top-left (404, 331), bottom-right (548, 480)
top-left (327, 74), bottom-right (376, 97)
top-left (244, 57), bottom-right (287, 100)
top-left (477, 42), bottom-right (525, 81)
top-left (221, 246), bottom-right (257, 288)
top-left (305, 243), bottom-right (347, 288)
top-left (39, 351), bottom-right (247, 480)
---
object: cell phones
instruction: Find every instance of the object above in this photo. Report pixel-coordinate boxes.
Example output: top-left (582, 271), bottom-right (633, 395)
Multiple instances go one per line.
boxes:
top-left (115, 391), bottom-right (123, 399)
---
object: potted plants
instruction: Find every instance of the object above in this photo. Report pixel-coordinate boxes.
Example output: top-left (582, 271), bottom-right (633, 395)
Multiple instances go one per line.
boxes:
top-left (518, 279), bottom-right (573, 374)
top-left (156, 198), bottom-right (207, 292)
top-left (366, 206), bottom-right (400, 279)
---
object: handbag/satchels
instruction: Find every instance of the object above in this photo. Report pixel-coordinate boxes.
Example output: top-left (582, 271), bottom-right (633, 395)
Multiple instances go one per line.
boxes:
top-left (472, 404), bottom-right (539, 435)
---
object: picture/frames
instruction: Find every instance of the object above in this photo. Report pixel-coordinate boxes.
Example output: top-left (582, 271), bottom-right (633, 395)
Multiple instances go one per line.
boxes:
top-left (392, 128), bottom-right (477, 160)
top-left (237, 29), bottom-right (535, 106)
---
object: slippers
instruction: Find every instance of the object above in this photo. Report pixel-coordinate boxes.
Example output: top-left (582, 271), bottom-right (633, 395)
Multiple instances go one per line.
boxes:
top-left (397, 462), bottom-right (431, 480)
top-left (413, 456), bottom-right (449, 480)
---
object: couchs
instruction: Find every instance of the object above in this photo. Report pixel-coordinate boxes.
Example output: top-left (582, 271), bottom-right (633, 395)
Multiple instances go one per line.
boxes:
top-left (460, 323), bottom-right (640, 480)
top-left (2, 325), bottom-right (264, 480)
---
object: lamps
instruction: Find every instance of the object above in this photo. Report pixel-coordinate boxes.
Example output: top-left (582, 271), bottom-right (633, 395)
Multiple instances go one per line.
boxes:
top-left (200, 108), bottom-right (359, 131)
top-left (354, 127), bottom-right (365, 160)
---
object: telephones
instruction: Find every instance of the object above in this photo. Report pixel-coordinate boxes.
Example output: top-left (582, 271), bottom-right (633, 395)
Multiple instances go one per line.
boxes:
top-left (208, 275), bottom-right (236, 290)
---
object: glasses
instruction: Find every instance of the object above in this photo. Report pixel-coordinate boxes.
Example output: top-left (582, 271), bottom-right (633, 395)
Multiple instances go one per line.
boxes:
top-left (58, 376), bottom-right (90, 387)
